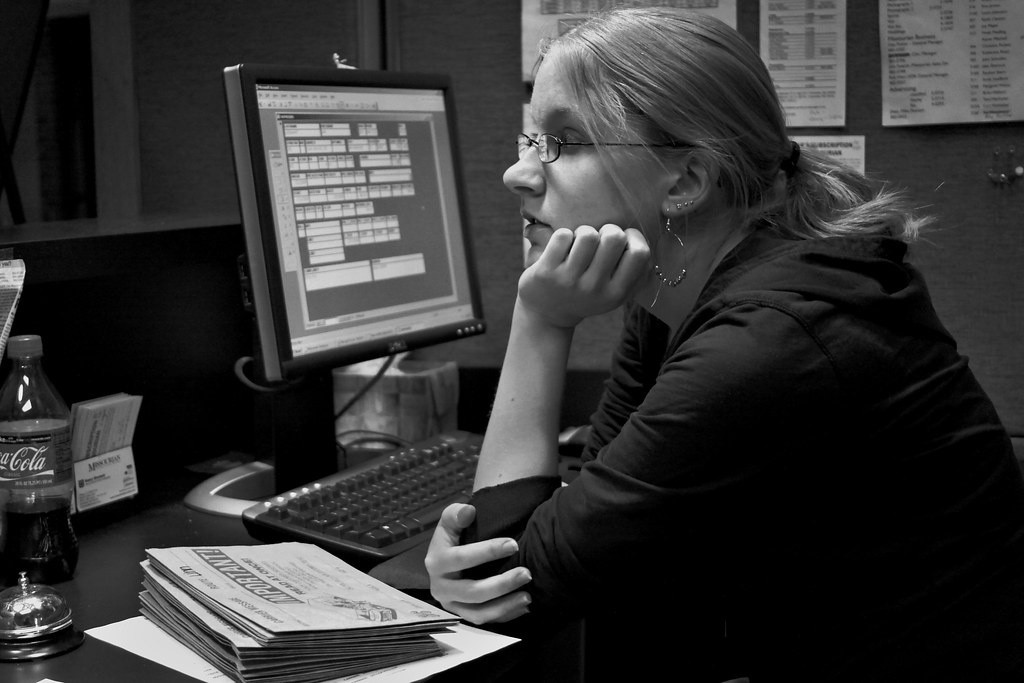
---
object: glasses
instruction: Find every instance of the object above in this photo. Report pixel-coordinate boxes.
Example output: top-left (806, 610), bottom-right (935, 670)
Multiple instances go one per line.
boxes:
top-left (515, 131), bottom-right (722, 189)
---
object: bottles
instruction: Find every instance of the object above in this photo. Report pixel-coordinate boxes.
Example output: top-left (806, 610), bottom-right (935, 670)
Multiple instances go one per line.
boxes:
top-left (0, 333), bottom-right (77, 660)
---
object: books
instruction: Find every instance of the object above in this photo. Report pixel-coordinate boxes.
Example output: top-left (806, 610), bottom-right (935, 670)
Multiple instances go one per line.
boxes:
top-left (139, 541), bottom-right (462, 683)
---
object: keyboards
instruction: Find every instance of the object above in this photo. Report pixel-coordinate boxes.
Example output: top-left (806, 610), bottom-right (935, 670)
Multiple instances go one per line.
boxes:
top-left (242, 430), bottom-right (487, 569)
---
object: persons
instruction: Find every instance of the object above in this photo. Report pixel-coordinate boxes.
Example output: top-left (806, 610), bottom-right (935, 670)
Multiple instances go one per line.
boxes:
top-left (425, 9), bottom-right (1024, 683)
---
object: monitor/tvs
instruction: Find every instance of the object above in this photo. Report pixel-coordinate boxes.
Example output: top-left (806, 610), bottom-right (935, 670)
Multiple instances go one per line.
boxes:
top-left (181, 64), bottom-right (488, 517)
